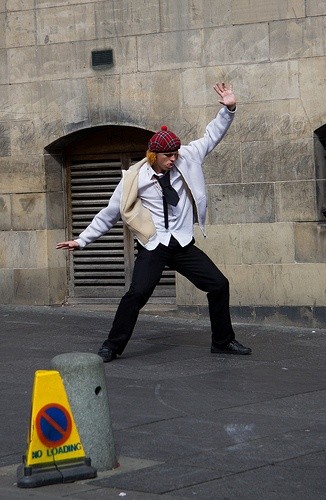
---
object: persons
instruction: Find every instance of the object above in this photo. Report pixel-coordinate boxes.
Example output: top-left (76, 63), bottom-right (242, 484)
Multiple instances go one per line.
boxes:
top-left (55, 81), bottom-right (252, 362)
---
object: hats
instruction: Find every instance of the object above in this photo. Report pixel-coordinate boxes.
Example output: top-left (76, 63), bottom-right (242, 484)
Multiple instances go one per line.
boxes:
top-left (149, 126), bottom-right (182, 153)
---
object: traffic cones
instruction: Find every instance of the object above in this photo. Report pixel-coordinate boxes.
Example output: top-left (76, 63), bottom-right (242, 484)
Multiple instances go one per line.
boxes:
top-left (16, 369), bottom-right (98, 490)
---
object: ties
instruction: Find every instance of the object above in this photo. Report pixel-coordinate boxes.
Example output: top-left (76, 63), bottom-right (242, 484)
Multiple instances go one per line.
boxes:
top-left (155, 172), bottom-right (180, 229)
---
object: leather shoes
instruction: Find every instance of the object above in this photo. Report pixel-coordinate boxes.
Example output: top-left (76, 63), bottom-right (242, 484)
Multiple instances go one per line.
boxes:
top-left (97, 343), bottom-right (116, 363)
top-left (211, 340), bottom-right (252, 354)
